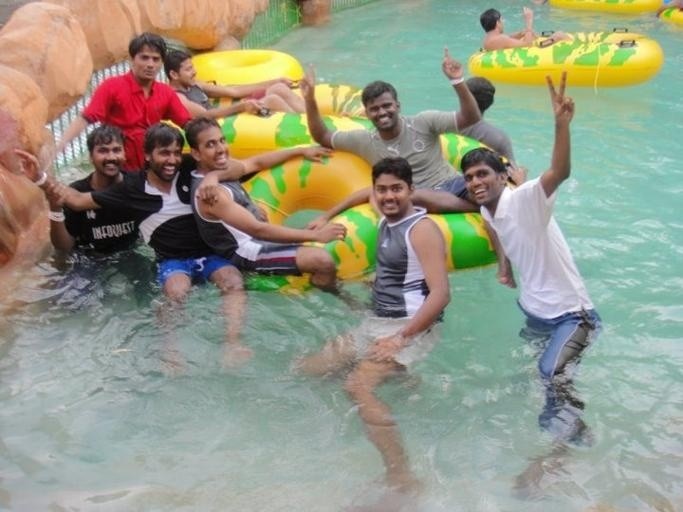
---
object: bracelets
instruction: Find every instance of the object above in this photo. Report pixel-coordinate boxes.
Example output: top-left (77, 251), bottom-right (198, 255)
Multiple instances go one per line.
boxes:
top-left (451, 77), bottom-right (463, 84)
top-left (34, 173), bottom-right (47, 185)
top-left (49, 211), bottom-right (65, 221)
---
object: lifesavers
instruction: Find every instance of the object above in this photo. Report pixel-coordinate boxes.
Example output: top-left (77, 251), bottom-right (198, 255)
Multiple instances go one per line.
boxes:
top-left (548, 0), bottom-right (663, 13)
top-left (238, 146), bottom-right (388, 295)
top-left (168, 49), bottom-right (306, 92)
top-left (422, 131), bottom-right (517, 273)
top-left (468, 27), bottom-right (664, 88)
top-left (161, 80), bottom-right (378, 162)
top-left (659, 7), bottom-right (682, 29)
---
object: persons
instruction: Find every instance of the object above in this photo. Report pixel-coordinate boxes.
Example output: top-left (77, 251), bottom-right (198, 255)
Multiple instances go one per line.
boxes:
top-left (45, 128), bottom-right (139, 250)
top-left (14, 123), bottom-right (246, 281)
top-left (61, 30), bottom-right (192, 176)
top-left (480, 5), bottom-right (573, 51)
top-left (301, 46), bottom-right (512, 284)
top-left (655, 0), bottom-right (683, 18)
top-left (463, 77), bottom-right (512, 159)
top-left (309, 158), bottom-right (451, 334)
top-left (165, 50), bottom-right (348, 118)
top-left (462, 73), bottom-right (595, 374)
top-left (187, 116), bottom-right (348, 285)
